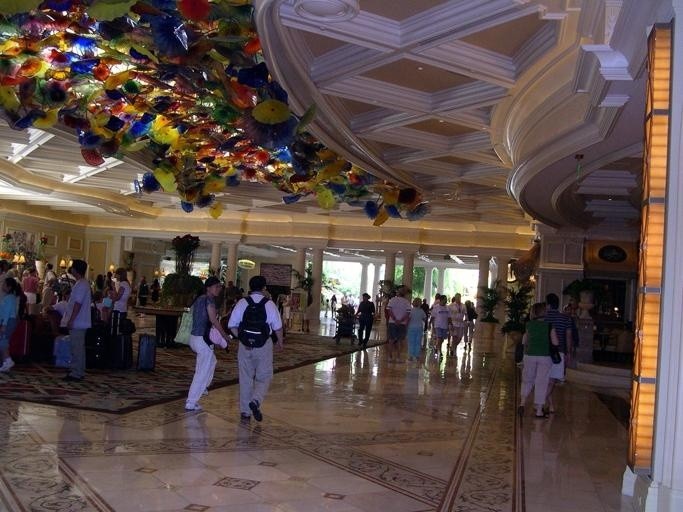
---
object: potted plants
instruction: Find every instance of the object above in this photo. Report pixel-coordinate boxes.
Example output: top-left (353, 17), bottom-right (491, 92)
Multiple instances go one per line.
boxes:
top-left (162, 234), bottom-right (204, 307)
top-left (477, 280), bottom-right (505, 340)
top-left (502, 285), bottom-right (535, 353)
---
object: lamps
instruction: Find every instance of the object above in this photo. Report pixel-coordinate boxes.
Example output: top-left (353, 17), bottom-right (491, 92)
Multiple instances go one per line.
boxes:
top-left (12, 254), bottom-right (26, 271)
top-left (59, 257), bottom-right (73, 272)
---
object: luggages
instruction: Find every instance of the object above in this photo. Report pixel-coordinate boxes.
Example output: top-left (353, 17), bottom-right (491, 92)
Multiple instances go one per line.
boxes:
top-left (9, 318), bottom-right (31, 358)
top-left (138, 334), bottom-right (157, 369)
top-left (85, 305), bottom-right (110, 369)
top-left (110, 308), bottom-right (133, 368)
top-left (52, 334), bottom-right (73, 370)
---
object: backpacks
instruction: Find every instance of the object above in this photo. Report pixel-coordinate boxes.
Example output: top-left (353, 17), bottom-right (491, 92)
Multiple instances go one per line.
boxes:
top-left (237, 295), bottom-right (270, 348)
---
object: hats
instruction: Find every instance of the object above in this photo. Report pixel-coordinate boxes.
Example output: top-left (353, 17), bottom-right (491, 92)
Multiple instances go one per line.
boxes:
top-left (205, 277), bottom-right (220, 286)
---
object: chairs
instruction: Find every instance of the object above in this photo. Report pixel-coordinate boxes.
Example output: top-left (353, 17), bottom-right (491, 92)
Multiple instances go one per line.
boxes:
top-left (47, 310), bottom-right (72, 367)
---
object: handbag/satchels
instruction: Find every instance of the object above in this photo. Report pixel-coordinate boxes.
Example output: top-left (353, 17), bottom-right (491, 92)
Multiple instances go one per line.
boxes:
top-left (548, 322), bottom-right (562, 364)
top-left (173, 307), bottom-right (194, 346)
top-left (204, 320), bottom-right (228, 352)
top-left (107, 319), bottom-right (135, 336)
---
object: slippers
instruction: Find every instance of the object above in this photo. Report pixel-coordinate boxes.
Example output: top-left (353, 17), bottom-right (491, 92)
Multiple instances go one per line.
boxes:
top-left (535, 413), bottom-right (549, 418)
top-left (517, 405), bottom-right (524, 417)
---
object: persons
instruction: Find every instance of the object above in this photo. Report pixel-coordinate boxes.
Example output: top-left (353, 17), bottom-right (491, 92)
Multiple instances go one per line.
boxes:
top-left (275, 288), bottom-right (479, 364)
top-left (333, 349), bottom-right (475, 420)
top-left (226, 275), bottom-right (284, 422)
top-left (561, 295), bottom-right (581, 318)
top-left (516, 302), bottom-right (560, 418)
top-left (531, 290), bottom-right (574, 414)
top-left (0, 251), bottom-right (161, 381)
top-left (182, 273), bottom-right (233, 411)
top-left (513, 310), bottom-right (529, 366)
top-left (0, 396), bottom-right (124, 488)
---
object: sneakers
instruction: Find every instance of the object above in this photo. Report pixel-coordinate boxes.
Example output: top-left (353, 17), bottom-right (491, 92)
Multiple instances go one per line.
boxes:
top-left (0, 358), bottom-right (15, 371)
top-left (248, 399), bottom-right (262, 421)
top-left (201, 388), bottom-right (209, 396)
top-left (241, 411), bottom-right (250, 419)
top-left (185, 401), bottom-right (202, 411)
